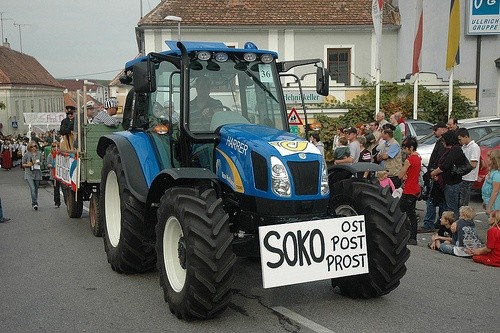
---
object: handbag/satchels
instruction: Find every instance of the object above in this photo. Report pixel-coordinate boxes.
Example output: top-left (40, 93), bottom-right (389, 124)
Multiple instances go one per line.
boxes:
top-left (450, 152), bottom-right (474, 177)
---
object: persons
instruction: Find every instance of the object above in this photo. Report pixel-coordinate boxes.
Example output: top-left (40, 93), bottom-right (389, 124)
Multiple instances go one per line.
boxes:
top-left (93, 99), bottom-right (119, 127)
top-left (0, 137), bottom-right (27, 171)
top-left (470, 210), bottom-right (500, 267)
top-left (417, 117), bottom-right (480, 234)
top-left (481, 150), bottom-right (500, 215)
top-left (82, 101), bottom-right (96, 124)
top-left (60, 105), bottom-right (76, 135)
top-left (22, 141), bottom-right (44, 210)
top-left (189, 76), bottom-right (223, 126)
top-left (440, 206), bottom-right (484, 256)
top-left (397, 137), bottom-right (421, 245)
top-left (429, 211), bottom-right (456, 250)
top-left (332, 111), bottom-right (406, 194)
top-left (154, 101), bottom-right (180, 123)
top-left (311, 134), bottom-right (324, 156)
top-left (31, 129), bottom-right (62, 151)
top-left (48, 142), bottom-right (67, 207)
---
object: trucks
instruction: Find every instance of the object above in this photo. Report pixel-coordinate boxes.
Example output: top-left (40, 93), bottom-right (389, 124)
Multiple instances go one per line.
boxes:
top-left (50, 42), bottom-right (411, 324)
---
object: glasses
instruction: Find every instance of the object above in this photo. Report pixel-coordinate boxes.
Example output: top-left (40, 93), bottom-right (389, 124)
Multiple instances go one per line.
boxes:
top-left (29, 144), bottom-right (37, 148)
top-left (66, 112), bottom-right (74, 115)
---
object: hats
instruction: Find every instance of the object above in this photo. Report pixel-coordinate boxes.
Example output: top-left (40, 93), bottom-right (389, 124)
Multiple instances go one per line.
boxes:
top-left (429, 121), bottom-right (446, 130)
top-left (103, 98), bottom-right (119, 109)
top-left (343, 127), bottom-right (357, 134)
top-left (64, 105), bottom-right (78, 114)
top-left (81, 100), bottom-right (95, 109)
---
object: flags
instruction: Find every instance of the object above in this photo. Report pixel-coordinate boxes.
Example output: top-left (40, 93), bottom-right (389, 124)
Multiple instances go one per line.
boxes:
top-left (372, 0), bottom-right (383, 72)
top-left (446, 0), bottom-right (460, 71)
top-left (413, 0), bottom-right (423, 73)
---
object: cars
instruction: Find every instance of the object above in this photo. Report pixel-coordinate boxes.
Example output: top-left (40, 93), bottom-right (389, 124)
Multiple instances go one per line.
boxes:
top-left (404, 115), bottom-right (500, 201)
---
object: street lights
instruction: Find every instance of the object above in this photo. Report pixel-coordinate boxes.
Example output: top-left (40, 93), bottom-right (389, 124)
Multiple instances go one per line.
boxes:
top-left (163, 15), bottom-right (183, 41)
top-left (85, 82), bottom-right (105, 108)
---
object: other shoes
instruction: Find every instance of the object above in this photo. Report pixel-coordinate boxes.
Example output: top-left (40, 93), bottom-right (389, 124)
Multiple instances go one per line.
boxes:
top-left (0, 216), bottom-right (12, 224)
top-left (418, 223), bottom-right (440, 234)
top-left (33, 204), bottom-right (39, 210)
top-left (54, 204), bottom-right (60, 208)
